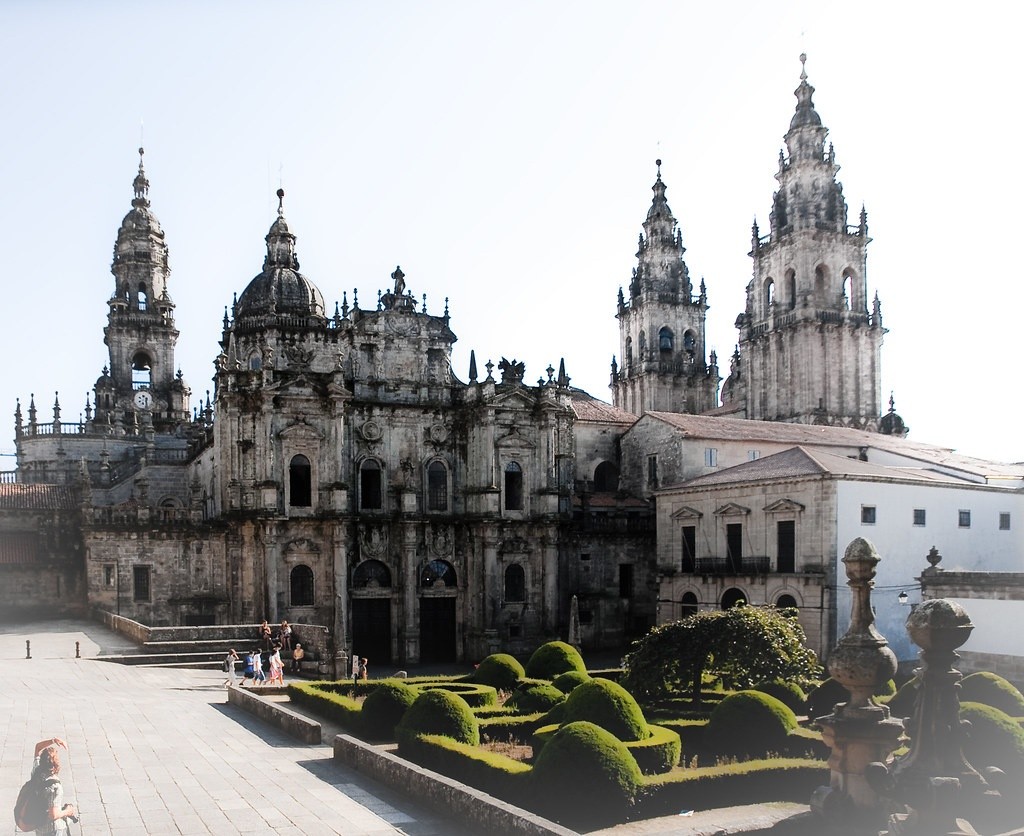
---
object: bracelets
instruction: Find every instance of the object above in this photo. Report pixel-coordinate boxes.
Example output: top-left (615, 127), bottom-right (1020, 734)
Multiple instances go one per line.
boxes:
top-left (52, 737), bottom-right (56, 744)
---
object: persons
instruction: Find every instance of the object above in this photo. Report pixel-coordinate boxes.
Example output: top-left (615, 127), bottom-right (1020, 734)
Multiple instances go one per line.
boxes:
top-left (290, 643), bottom-right (304, 672)
top-left (222, 649), bottom-right (239, 688)
top-left (239, 643), bottom-right (285, 685)
top-left (259, 619), bottom-right (276, 652)
top-left (31, 738), bottom-right (75, 836)
top-left (276, 620), bottom-right (293, 651)
top-left (358, 658), bottom-right (367, 680)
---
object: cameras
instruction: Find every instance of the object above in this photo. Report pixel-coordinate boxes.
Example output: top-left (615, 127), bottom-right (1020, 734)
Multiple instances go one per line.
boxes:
top-left (62, 803), bottom-right (78, 823)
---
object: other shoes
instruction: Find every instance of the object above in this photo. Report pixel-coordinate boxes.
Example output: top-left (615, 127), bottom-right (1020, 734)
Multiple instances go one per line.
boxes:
top-left (223, 683), bottom-right (226, 688)
top-left (290, 667), bottom-right (293, 671)
top-left (297, 668), bottom-right (300, 672)
top-left (267, 649), bottom-right (269, 651)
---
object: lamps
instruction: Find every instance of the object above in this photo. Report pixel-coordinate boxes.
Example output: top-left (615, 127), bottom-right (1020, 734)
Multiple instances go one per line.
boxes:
top-left (898, 591), bottom-right (908, 605)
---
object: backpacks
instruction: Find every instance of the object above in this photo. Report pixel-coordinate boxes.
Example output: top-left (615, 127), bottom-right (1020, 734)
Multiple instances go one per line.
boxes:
top-left (14, 770), bottom-right (61, 832)
top-left (242, 659), bottom-right (248, 669)
top-left (222, 659), bottom-right (228, 672)
